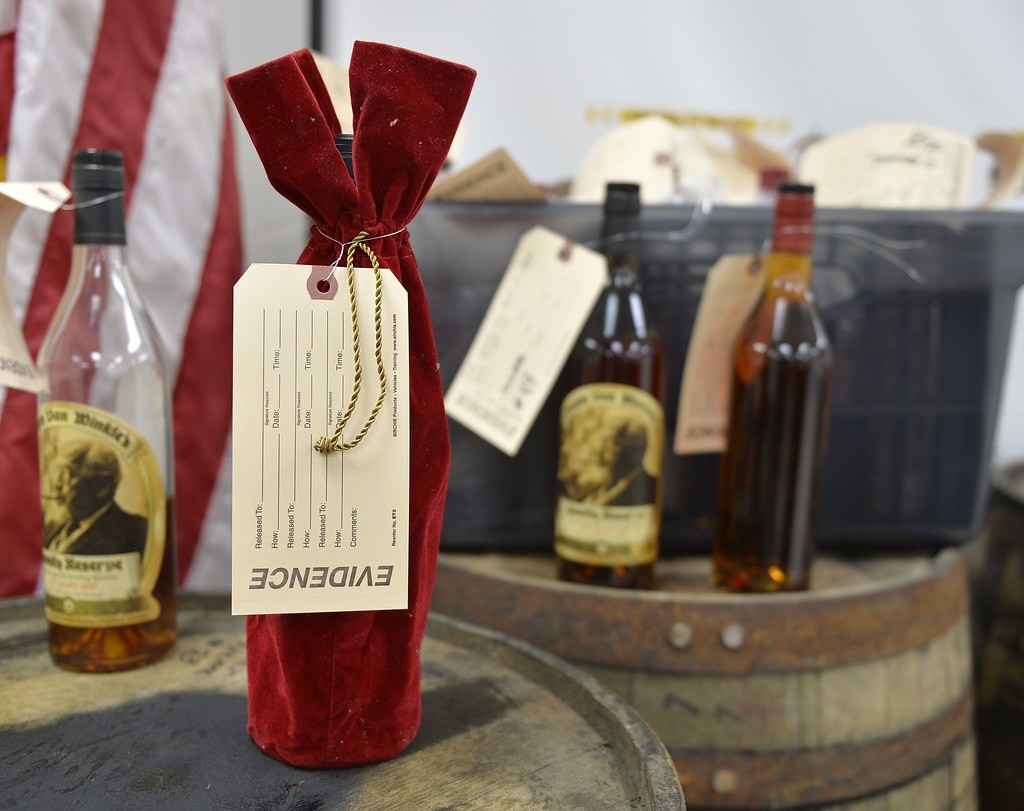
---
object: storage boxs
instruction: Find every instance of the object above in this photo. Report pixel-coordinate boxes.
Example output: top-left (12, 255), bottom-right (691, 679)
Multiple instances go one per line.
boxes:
top-left (405, 202), bottom-right (1024, 556)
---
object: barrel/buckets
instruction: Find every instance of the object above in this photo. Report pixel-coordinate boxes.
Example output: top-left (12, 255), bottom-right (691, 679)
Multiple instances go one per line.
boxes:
top-left (387, 196), bottom-right (1024, 558)
top-left (435, 550), bottom-right (980, 810)
top-left (0, 611), bottom-right (686, 810)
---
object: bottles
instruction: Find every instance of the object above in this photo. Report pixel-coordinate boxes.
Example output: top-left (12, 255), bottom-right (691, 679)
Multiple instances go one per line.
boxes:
top-left (39, 150), bottom-right (177, 673)
top-left (711, 184), bottom-right (834, 592)
top-left (554, 184), bottom-right (667, 588)
